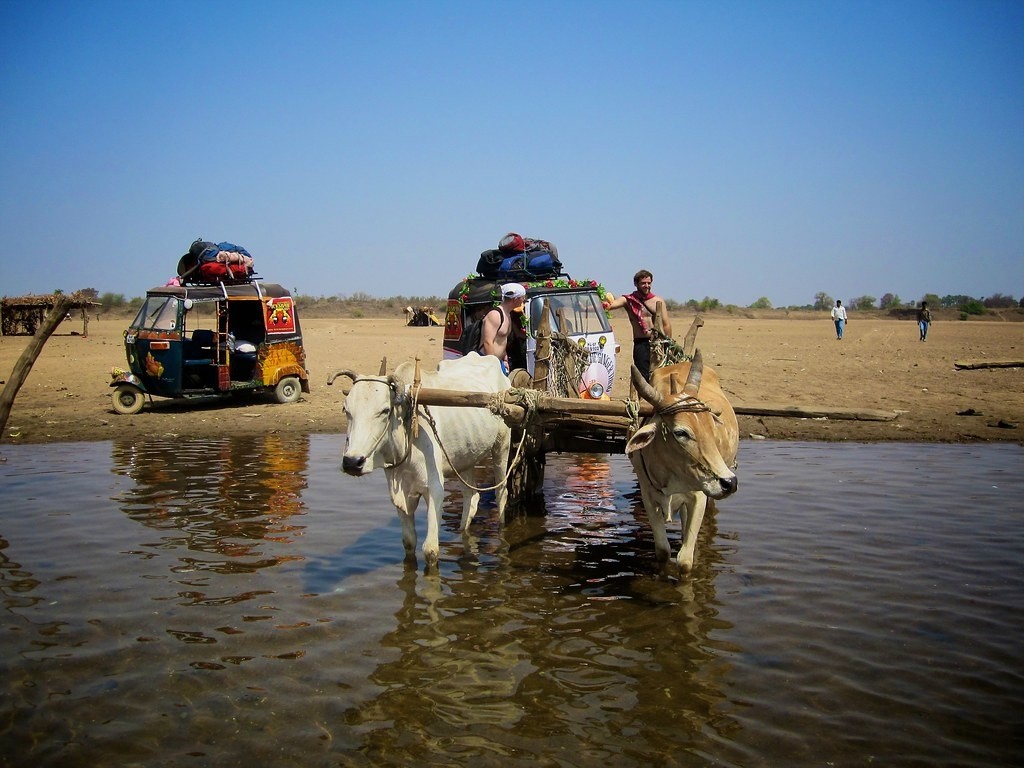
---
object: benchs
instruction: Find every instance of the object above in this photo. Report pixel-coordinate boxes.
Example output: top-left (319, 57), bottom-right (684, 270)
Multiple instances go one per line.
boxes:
top-left (219, 334), bottom-right (259, 359)
top-left (184, 329), bottom-right (216, 365)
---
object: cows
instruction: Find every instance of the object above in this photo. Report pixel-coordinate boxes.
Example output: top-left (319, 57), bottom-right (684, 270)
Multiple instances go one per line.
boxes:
top-left (623, 347), bottom-right (741, 576)
top-left (325, 349), bottom-right (513, 567)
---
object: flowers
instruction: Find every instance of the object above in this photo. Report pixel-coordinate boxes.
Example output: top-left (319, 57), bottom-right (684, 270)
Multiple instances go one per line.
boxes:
top-left (458, 274), bottom-right (479, 307)
top-left (517, 279), bottom-right (614, 336)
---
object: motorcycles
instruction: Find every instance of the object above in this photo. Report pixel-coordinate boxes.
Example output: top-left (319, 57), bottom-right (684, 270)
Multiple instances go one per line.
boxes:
top-left (441, 279), bottom-right (621, 402)
top-left (109, 283), bottom-right (310, 415)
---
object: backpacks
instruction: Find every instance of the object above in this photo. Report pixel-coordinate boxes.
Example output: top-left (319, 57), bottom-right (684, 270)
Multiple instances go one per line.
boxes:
top-left (459, 307), bottom-right (504, 356)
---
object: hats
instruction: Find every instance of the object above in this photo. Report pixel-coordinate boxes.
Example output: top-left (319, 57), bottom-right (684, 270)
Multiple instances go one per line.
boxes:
top-left (501, 283), bottom-right (527, 300)
